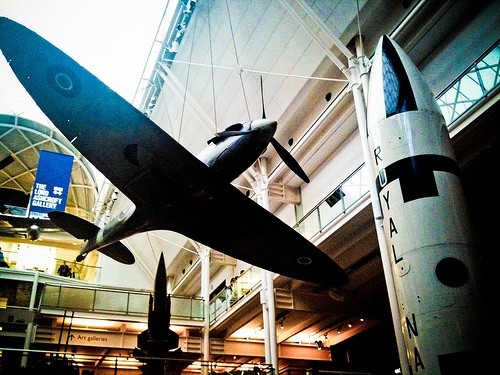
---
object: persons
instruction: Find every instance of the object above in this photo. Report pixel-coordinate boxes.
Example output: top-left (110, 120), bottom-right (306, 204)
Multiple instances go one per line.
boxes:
top-left (57, 260), bottom-right (70, 276)
top-left (0, 246), bottom-right (4, 264)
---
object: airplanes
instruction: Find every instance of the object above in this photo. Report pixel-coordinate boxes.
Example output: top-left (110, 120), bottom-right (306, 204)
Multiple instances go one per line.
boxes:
top-left (1, 16), bottom-right (349, 289)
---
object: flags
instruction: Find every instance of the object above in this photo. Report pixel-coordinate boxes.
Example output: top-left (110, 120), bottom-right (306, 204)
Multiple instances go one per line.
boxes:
top-left (28, 150), bottom-right (75, 219)
